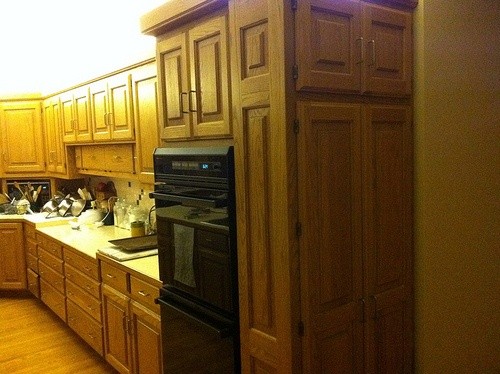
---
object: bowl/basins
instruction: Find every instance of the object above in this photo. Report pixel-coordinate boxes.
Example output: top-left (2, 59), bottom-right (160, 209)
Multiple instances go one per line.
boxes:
top-left (40, 198), bottom-right (86, 219)
top-left (9, 204), bottom-right (27, 215)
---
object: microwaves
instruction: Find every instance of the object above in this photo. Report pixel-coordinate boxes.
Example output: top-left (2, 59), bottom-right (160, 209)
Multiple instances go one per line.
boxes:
top-left (7, 182), bottom-right (50, 213)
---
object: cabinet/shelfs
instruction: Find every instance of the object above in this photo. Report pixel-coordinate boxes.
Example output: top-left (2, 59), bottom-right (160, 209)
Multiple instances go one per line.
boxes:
top-left (0, 56), bottom-right (155, 185)
top-left (143, 0), bottom-right (417, 374)
top-left (0, 219), bottom-right (162, 374)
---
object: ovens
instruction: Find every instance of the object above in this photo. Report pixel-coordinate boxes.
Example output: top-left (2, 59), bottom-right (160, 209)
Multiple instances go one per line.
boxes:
top-left (149, 147), bottom-right (241, 374)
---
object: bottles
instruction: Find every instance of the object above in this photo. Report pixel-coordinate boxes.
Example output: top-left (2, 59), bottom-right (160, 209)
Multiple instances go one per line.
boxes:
top-left (113, 198), bottom-right (128, 228)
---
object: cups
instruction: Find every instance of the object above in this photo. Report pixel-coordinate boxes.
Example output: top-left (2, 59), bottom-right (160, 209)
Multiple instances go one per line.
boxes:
top-left (104, 212), bottom-right (114, 225)
top-left (130, 221), bottom-right (145, 238)
